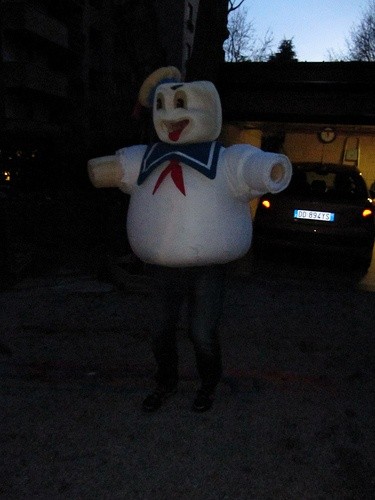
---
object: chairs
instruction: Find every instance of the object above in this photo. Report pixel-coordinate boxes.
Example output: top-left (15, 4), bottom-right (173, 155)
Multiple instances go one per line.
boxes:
top-left (307, 179), bottom-right (325, 199)
top-left (327, 174), bottom-right (358, 200)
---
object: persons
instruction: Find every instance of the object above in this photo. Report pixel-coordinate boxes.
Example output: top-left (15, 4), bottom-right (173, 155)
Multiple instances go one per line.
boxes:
top-left (88, 62), bottom-right (294, 415)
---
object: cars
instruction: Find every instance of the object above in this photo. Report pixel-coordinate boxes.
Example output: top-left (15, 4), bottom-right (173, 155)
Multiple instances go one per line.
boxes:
top-left (253, 161), bottom-right (375, 271)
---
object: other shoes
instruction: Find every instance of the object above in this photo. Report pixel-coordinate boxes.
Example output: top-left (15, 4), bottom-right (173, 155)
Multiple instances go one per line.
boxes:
top-left (194, 386), bottom-right (215, 411)
top-left (143, 382), bottom-right (177, 412)
